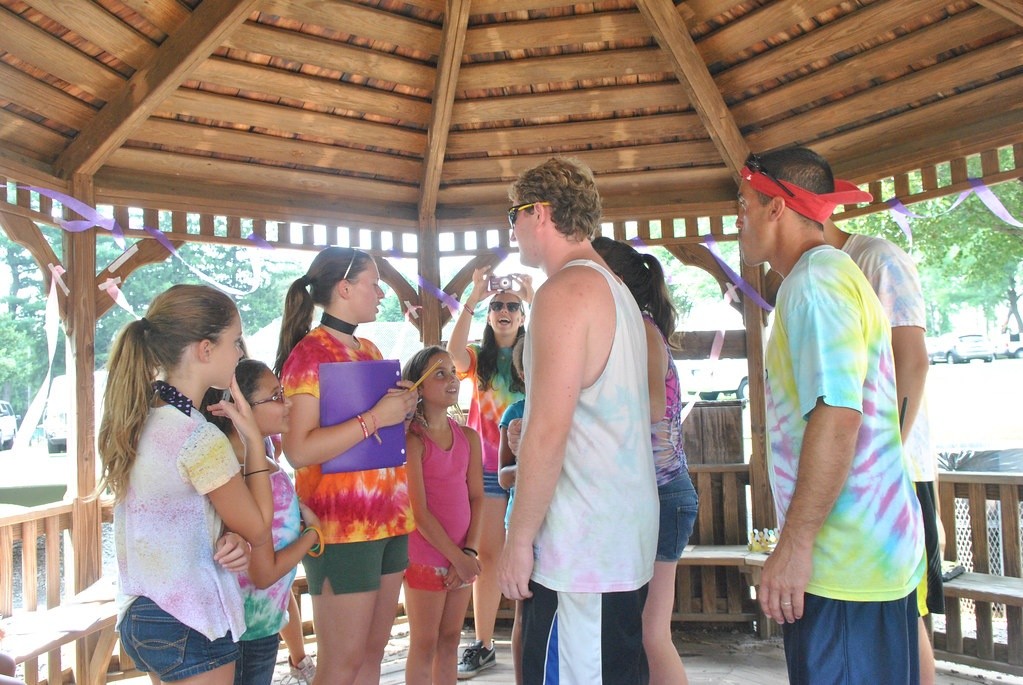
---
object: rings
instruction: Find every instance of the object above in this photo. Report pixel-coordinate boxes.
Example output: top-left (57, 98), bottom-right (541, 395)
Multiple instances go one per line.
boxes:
top-left (781, 601), bottom-right (792, 606)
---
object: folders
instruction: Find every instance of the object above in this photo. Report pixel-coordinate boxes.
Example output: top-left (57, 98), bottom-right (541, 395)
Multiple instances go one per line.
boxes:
top-left (318, 359), bottom-right (407, 474)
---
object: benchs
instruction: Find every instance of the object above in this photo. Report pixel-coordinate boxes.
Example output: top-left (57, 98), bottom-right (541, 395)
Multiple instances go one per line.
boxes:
top-left (668, 543), bottom-right (1023, 607)
top-left (0, 593), bottom-right (118, 685)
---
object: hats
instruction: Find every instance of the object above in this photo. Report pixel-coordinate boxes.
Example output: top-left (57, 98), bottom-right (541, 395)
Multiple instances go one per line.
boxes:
top-left (738, 165), bottom-right (873, 226)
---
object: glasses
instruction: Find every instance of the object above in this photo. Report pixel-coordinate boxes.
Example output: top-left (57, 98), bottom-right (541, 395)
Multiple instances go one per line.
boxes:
top-left (247, 387), bottom-right (286, 409)
top-left (490, 301), bottom-right (523, 315)
top-left (343, 247), bottom-right (365, 279)
top-left (742, 150), bottom-right (796, 200)
top-left (506, 202), bottom-right (551, 229)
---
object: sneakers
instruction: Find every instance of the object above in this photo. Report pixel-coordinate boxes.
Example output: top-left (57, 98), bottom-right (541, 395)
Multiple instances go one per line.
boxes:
top-left (457, 636), bottom-right (496, 679)
top-left (279, 653), bottom-right (316, 685)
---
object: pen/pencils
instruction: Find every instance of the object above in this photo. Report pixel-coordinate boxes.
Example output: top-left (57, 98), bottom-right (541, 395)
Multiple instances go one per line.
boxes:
top-left (409, 359), bottom-right (443, 390)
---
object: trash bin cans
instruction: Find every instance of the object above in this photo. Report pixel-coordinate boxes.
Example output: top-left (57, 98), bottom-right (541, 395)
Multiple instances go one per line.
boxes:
top-left (936, 448), bottom-right (1023, 620)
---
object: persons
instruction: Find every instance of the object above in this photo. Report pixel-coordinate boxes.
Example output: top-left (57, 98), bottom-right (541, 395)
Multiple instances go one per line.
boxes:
top-left (401, 346), bottom-right (484, 685)
top-left (198, 359), bottom-right (324, 685)
top-left (239, 338), bottom-right (316, 685)
top-left (446, 266), bottom-right (535, 680)
top-left (496, 156), bottom-right (660, 685)
top-left (80, 284), bottom-right (273, 685)
top-left (273, 247), bottom-right (422, 685)
top-left (591, 237), bottom-right (699, 685)
top-left (736, 147), bottom-right (945, 685)
top-left (498, 336), bottom-right (524, 685)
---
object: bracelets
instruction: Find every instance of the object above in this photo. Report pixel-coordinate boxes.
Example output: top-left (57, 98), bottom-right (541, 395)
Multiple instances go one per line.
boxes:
top-left (223, 532), bottom-right (251, 552)
top-left (463, 547), bottom-right (478, 557)
top-left (368, 409), bottom-right (382, 445)
top-left (405, 398), bottom-right (422, 420)
top-left (357, 415), bottom-right (369, 440)
top-left (243, 469), bottom-right (269, 476)
top-left (464, 304), bottom-right (474, 315)
top-left (301, 526), bottom-right (324, 557)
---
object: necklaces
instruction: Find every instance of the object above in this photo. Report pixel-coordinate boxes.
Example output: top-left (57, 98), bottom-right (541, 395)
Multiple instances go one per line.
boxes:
top-left (321, 312), bottom-right (358, 335)
